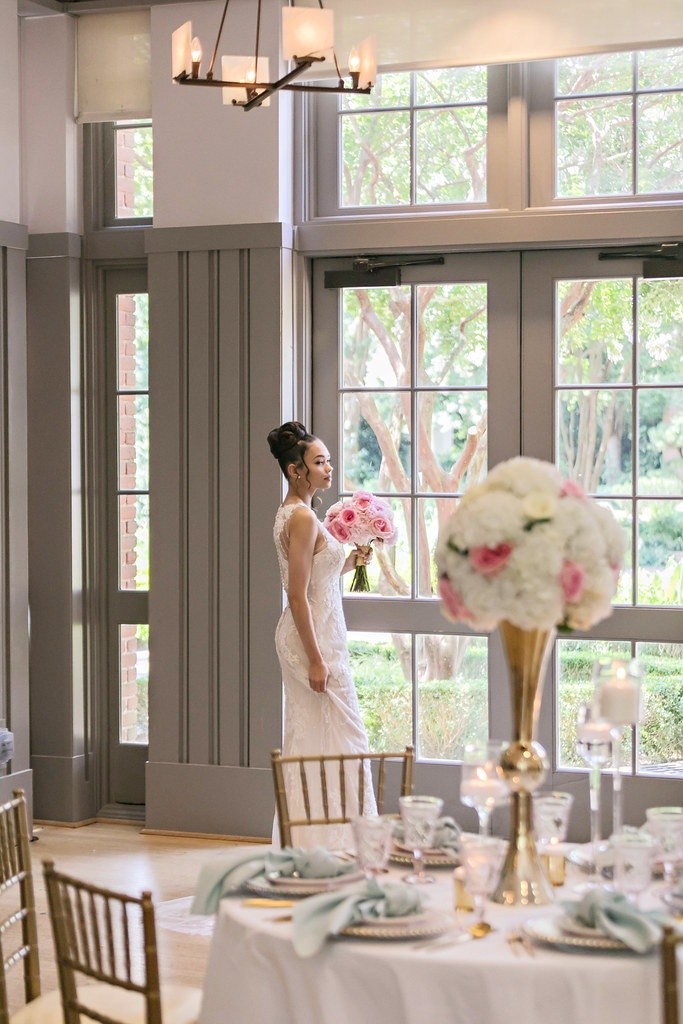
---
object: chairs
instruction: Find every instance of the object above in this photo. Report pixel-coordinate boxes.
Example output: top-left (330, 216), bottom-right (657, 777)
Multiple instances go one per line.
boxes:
top-left (43, 861), bottom-right (164, 1024)
top-left (0, 789), bottom-right (199, 1024)
top-left (273, 748), bottom-right (422, 845)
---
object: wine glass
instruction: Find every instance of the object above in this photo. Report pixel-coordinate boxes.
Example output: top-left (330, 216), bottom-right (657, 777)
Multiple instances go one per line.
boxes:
top-left (613, 803), bottom-right (683, 902)
top-left (456, 838), bottom-right (509, 938)
top-left (458, 740), bottom-right (518, 841)
top-left (397, 795), bottom-right (445, 882)
top-left (348, 814), bottom-right (394, 893)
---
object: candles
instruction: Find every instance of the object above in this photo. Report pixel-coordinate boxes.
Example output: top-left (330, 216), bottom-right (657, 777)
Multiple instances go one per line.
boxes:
top-left (466, 767), bottom-right (507, 800)
top-left (604, 668), bottom-right (638, 723)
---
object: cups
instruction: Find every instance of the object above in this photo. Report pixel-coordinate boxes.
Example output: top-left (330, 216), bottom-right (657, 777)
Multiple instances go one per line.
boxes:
top-left (575, 710), bottom-right (621, 767)
top-left (593, 654), bottom-right (643, 724)
top-left (530, 792), bottom-right (574, 849)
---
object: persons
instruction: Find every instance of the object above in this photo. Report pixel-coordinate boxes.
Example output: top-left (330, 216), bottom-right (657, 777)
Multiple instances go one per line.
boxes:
top-left (268, 422), bottom-right (379, 848)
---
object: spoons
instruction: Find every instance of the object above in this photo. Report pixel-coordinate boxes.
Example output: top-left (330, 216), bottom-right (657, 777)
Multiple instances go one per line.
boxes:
top-left (421, 920), bottom-right (491, 953)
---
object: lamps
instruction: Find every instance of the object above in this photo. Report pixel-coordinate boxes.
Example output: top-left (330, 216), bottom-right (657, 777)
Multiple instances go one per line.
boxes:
top-left (173, 0), bottom-right (380, 114)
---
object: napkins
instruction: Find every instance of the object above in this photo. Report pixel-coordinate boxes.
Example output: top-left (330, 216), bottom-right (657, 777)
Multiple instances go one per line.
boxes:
top-left (387, 817), bottom-right (455, 849)
top-left (286, 872), bottom-right (427, 959)
top-left (195, 846), bottom-right (350, 920)
top-left (563, 883), bottom-right (671, 953)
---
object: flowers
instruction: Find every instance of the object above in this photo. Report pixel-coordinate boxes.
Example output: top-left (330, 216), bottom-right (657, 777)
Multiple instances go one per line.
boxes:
top-left (432, 461), bottom-right (626, 636)
top-left (323, 488), bottom-right (403, 597)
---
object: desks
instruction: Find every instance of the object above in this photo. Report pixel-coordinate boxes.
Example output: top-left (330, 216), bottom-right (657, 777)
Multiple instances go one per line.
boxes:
top-left (197, 831), bottom-right (682, 1024)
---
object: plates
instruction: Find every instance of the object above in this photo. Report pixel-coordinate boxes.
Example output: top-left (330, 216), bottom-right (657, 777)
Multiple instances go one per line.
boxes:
top-left (522, 899), bottom-right (682, 955)
top-left (567, 837), bottom-right (610, 873)
top-left (318, 903), bottom-right (454, 938)
top-left (243, 854), bottom-right (367, 899)
top-left (387, 840), bottom-right (459, 868)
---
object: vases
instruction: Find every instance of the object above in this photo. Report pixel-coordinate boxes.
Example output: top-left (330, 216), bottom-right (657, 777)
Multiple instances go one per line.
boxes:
top-left (487, 617), bottom-right (557, 908)
